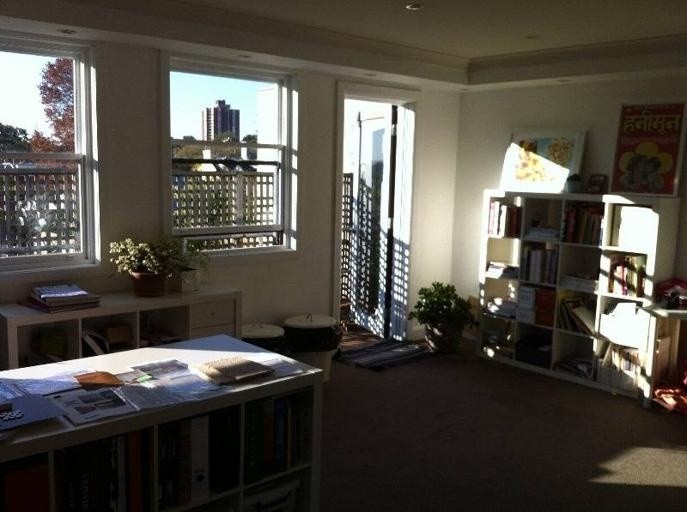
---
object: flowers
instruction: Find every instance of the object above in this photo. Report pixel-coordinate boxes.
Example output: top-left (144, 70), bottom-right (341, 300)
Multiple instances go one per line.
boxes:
top-left (109, 234), bottom-right (164, 274)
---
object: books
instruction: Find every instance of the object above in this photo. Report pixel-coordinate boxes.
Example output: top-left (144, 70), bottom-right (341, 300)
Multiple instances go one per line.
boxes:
top-left (487, 199), bottom-right (645, 393)
top-left (55, 392), bottom-right (310, 512)
top-left (16, 281), bottom-right (101, 314)
top-left (82, 318), bottom-right (109, 355)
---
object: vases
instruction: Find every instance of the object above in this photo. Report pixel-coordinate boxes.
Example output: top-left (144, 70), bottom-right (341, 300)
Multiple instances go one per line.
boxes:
top-left (128, 267), bottom-right (166, 297)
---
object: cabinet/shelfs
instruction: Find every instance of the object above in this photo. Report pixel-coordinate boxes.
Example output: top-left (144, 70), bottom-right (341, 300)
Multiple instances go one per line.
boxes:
top-left (1, 334), bottom-right (328, 512)
top-left (1, 283), bottom-right (244, 368)
top-left (476, 185), bottom-right (680, 408)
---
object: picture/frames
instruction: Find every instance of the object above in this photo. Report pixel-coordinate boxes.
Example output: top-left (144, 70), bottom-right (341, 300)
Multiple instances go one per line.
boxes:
top-left (608, 103), bottom-right (685, 196)
top-left (498, 128), bottom-right (586, 194)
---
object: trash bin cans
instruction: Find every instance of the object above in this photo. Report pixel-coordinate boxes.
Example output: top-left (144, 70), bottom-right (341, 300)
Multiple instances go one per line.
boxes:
top-left (240, 319), bottom-right (284, 351)
top-left (282, 311), bottom-right (340, 385)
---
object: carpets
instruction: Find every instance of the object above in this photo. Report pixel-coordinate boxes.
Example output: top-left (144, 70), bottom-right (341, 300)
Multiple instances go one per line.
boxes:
top-left (336, 337), bottom-right (429, 373)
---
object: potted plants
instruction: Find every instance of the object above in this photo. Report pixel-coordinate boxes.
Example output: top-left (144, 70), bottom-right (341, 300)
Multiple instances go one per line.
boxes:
top-left (566, 173), bottom-right (584, 194)
top-left (159, 235), bottom-right (211, 293)
top-left (410, 282), bottom-right (479, 359)
